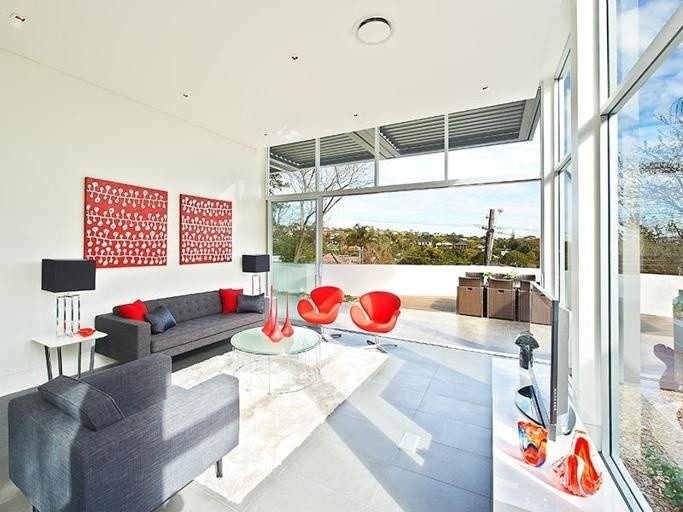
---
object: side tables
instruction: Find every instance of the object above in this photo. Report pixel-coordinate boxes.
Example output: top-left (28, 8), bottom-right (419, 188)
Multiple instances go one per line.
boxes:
top-left (30, 329), bottom-right (110, 380)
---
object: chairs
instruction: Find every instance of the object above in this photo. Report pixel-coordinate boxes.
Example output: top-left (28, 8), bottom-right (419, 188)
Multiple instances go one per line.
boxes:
top-left (457, 270), bottom-right (550, 325)
top-left (297, 286), bottom-right (402, 355)
top-left (1, 350), bottom-right (243, 512)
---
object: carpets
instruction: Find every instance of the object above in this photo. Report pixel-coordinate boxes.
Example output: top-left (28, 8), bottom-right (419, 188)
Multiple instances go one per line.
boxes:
top-left (168, 340), bottom-right (390, 503)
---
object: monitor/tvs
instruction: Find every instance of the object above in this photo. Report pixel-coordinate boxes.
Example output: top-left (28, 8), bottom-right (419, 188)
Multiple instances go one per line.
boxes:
top-left (515, 281), bottom-right (576, 441)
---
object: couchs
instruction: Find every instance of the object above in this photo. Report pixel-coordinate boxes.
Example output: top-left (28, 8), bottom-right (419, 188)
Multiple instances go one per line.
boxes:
top-left (94, 287), bottom-right (270, 359)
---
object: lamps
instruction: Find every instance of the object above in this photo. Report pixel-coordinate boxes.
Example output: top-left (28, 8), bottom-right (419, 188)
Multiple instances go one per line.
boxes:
top-left (38, 257), bottom-right (95, 334)
top-left (242, 254), bottom-right (270, 294)
top-left (352, 16), bottom-right (396, 45)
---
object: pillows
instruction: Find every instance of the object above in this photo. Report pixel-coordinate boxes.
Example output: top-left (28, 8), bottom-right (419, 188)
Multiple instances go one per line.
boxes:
top-left (115, 299), bottom-right (177, 334)
top-left (217, 288), bottom-right (266, 314)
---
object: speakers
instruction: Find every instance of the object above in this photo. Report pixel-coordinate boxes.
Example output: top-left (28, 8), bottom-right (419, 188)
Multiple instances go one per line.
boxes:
top-left (242, 255), bottom-right (270, 272)
top-left (41, 259), bottom-right (95, 292)
